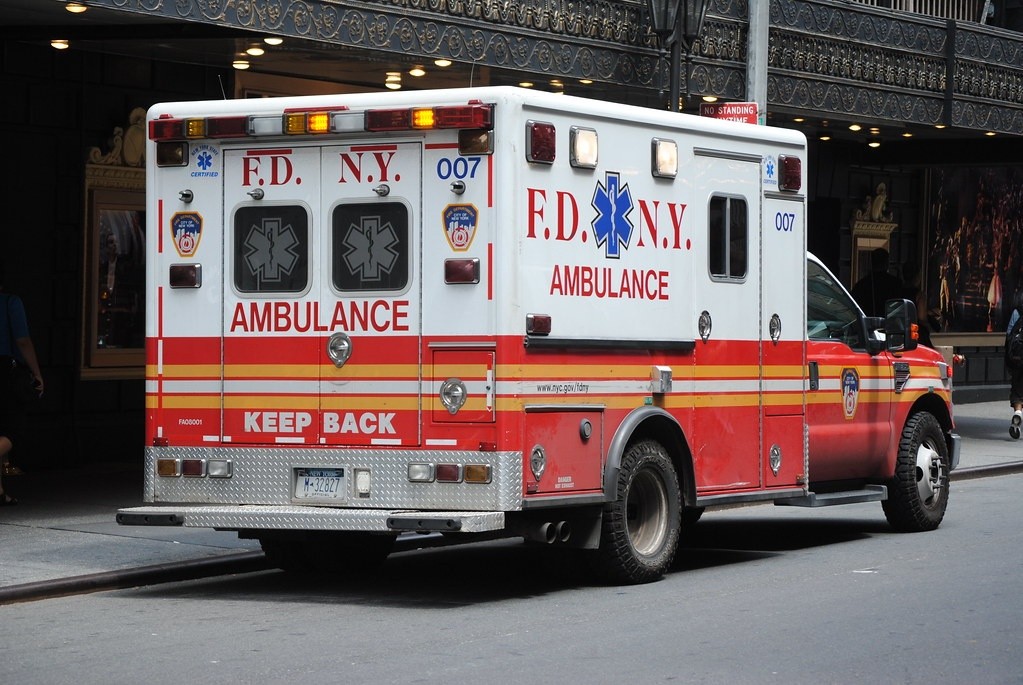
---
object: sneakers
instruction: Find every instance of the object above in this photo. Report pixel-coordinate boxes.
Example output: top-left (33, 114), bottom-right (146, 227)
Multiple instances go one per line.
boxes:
top-left (1009, 409), bottom-right (1023, 439)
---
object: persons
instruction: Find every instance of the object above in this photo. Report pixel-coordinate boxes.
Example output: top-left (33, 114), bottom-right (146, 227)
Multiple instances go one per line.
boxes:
top-left (98, 211), bottom-right (146, 349)
top-left (0, 291), bottom-right (45, 507)
top-left (1005, 303), bottom-right (1023, 441)
top-left (849, 248), bottom-right (928, 320)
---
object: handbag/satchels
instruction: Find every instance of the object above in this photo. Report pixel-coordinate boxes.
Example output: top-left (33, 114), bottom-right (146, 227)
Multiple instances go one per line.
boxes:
top-left (7, 355), bottom-right (32, 394)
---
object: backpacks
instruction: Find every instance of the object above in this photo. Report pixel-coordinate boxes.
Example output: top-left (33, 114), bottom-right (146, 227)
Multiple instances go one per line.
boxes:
top-left (1003, 306), bottom-right (1023, 370)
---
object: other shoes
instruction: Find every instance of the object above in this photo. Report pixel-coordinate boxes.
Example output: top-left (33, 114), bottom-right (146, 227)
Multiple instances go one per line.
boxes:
top-left (2, 463), bottom-right (25, 476)
top-left (0, 493), bottom-right (19, 506)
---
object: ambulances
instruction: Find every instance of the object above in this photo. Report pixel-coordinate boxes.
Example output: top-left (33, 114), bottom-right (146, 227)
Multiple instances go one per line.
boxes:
top-left (114, 81), bottom-right (965, 591)
